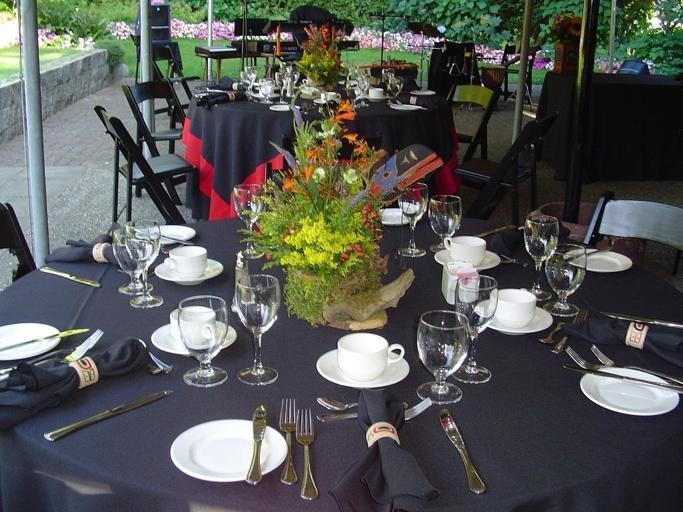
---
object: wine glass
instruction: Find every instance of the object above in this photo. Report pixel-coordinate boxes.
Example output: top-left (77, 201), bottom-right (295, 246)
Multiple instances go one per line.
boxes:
top-left (397, 183), bottom-right (427, 257)
top-left (178, 296), bottom-right (229, 386)
top-left (111, 229), bottom-right (154, 294)
top-left (125, 219), bottom-right (163, 307)
top-left (244, 65), bottom-right (258, 87)
top-left (543, 245), bottom-right (588, 316)
top-left (234, 186), bottom-right (264, 258)
top-left (451, 277), bottom-right (498, 385)
top-left (358, 75), bottom-right (369, 107)
top-left (428, 195), bottom-right (461, 253)
top-left (240, 71), bottom-right (252, 100)
top-left (275, 73), bottom-right (289, 104)
top-left (258, 79), bottom-right (275, 104)
top-left (523, 216), bottom-right (559, 301)
top-left (416, 310), bottom-right (469, 405)
top-left (236, 275), bottom-right (279, 385)
top-left (346, 84), bottom-right (358, 108)
top-left (383, 67), bottom-right (396, 91)
top-left (388, 77), bottom-right (403, 107)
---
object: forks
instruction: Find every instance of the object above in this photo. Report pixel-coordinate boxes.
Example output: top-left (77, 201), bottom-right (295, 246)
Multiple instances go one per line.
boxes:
top-left (296, 407), bottom-right (320, 501)
top-left (279, 397), bottom-right (299, 485)
top-left (565, 344), bottom-right (683, 390)
top-left (589, 344), bottom-right (683, 386)
top-left (315, 398), bottom-right (432, 422)
top-left (0, 328), bottom-right (105, 382)
top-left (552, 305), bottom-right (589, 355)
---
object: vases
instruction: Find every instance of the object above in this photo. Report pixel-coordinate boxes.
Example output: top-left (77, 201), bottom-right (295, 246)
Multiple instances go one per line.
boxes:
top-left (312, 78), bottom-right (338, 92)
top-left (303, 261), bottom-right (415, 331)
top-left (554, 42), bottom-right (579, 72)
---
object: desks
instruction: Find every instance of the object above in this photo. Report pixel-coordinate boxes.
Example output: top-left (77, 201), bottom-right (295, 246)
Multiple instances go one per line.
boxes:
top-left (532, 72), bottom-right (683, 184)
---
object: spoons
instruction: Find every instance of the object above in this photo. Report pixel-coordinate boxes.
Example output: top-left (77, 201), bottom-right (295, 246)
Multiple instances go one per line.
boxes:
top-left (538, 322), bottom-right (563, 344)
top-left (317, 397), bottom-right (408, 411)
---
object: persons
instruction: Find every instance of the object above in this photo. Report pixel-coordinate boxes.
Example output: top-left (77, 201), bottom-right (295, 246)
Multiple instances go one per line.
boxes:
top-left (289, 5), bottom-right (354, 48)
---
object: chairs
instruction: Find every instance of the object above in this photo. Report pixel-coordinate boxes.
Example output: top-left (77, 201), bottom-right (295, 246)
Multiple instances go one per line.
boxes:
top-left (121, 79), bottom-right (186, 206)
top-left (262, 131), bottom-right (382, 197)
top-left (585, 190), bottom-right (683, 292)
top-left (0, 202), bottom-right (37, 293)
top-left (447, 84), bottom-right (502, 166)
top-left (128, 32), bottom-right (141, 83)
top-left (137, 44), bottom-right (192, 114)
top-left (455, 110), bottom-right (559, 226)
top-left (93, 105), bottom-right (195, 225)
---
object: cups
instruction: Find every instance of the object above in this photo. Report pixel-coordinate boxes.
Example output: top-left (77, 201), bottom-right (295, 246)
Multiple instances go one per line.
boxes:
top-left (474, 299), bottom-right (553, 336)
top-left (154, 259), bottom-right (224, 286)
top-left (443, 236), bottom-right (487, 268)
top-left (363, 94), bottom-right (389, 102)
top-left (317, 348), bottom-right (409, 390)
top-left (434, 248), bottom-right (501, 271)
top-left (314, 98), bottom-right (342, 104)
top-left (369, 87), bottom-right (384, 97)
top-left (337, 332), bottom-right (405, 382)
top-left (489, 286), bottom-right (537, 330)
top-left (163, 246), bottom-right (208, 279)
top-left (152, 320), bottom-right (237, 356)
top-left (169, 304), bottom-right (217, 348)
top-left (302, 76), bottom-right (313, 85)
top-left (320, 92), bottom-right (342, 102)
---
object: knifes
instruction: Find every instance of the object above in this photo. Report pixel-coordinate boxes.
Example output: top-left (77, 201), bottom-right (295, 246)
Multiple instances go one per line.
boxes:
top-left (43, 389), bottom-right (172, 442)
top-left (600, 310), bottom-right (683, 328)
top-left (439, 409), bottom-right (487, 494)
top-left (160, 235), bottom-right (194, 246)
top-left (0, 329), bottom-right (90, 352)
top-left (563, 362), bottom-right (682, 390)
top-left (246, 406), bottom-right (266, 485)
top-left (41, 266), bottom-right (102, 288)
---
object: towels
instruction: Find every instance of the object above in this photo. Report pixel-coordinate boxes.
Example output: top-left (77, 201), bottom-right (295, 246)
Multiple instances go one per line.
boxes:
top-left (46, 235), bottom-right (125, 266)
top-left (208, 76), bottom-right (247, 91)
top-left (196, 92), bottom-right (246, 111)
top-left (328, 390), bottom-right (440, 512)
top-left (398, 94), bottom-right (447, 111)
top-left (490, 219), bottom-right (571, 257)
top-left (564, 311), bottom-right (683, 380)
top-left (0, 337), bottom-right (147, 431)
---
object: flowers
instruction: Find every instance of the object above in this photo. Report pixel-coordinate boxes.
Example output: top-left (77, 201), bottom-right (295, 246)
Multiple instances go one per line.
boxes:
top-left (292, 23), bottom-right (347, 84)
top-left (236, 98), bottom-right (443, 327)
top-left (551, 12), bottom-right (582, 42)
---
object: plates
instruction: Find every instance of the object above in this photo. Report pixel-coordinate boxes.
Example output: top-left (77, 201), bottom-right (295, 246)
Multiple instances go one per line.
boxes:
top-left (411, 90), bottom-right (436, 95)
top-left (170, 418), bottom-right (289, 482)
top-left (132, 225), bottom-right (196, 246)
top-left (269, 105), bottom-right (300, 111)
top-left (378, 208), bottom-right (421, 224)
top-left (562, 250), bottom-right (633, 272)
top-left (580, 367), bottom-right (679, 416)
top-left (253, 93), bottom-right (279, 99)
top-left (390, 105), bottom-right (421, 110)
top-left (0, 322), bottom-right (59, 360)
